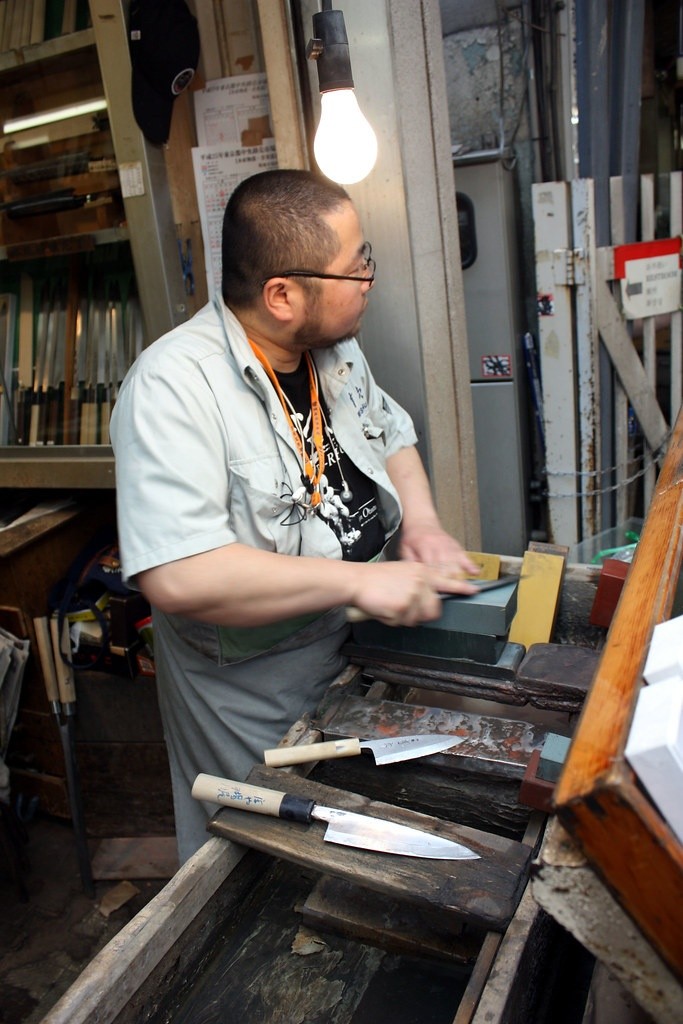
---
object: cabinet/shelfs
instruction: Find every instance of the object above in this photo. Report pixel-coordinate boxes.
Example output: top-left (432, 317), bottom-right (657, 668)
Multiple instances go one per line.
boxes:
top-left (1, 1), bottom-right (310, 493)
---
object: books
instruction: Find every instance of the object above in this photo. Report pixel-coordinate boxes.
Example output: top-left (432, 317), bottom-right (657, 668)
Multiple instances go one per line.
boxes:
top-left (0, 628), bottom-right (30, 763)
top-left (0, 2), bottom-right (144, 448)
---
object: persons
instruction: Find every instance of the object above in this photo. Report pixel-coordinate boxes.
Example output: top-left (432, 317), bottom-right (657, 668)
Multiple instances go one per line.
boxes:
top-left (112, 169), bottom-right (481, 868)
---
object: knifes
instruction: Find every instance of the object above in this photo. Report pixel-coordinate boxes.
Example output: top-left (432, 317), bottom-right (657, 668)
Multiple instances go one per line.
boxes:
top-left (263, 732), bottom-right (468, 766)
top-left (344, 574), bottom-right (532, 623)
top-left (2, 149), bottom-right (149, 905)
top-left (190, 772), bottom-right (483, 860)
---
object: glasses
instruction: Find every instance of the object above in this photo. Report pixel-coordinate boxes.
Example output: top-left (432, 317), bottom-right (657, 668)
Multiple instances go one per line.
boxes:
top-left (258, 243), bottom-right (376, 292)
